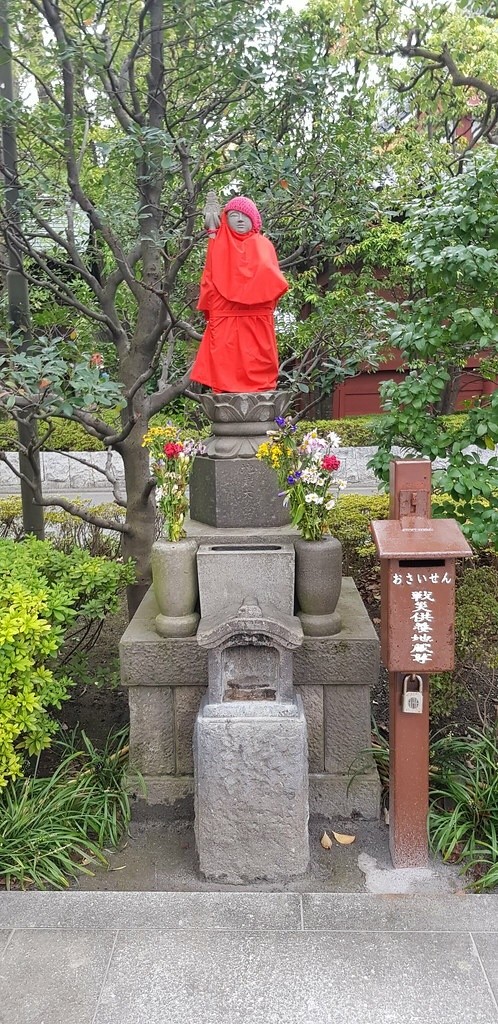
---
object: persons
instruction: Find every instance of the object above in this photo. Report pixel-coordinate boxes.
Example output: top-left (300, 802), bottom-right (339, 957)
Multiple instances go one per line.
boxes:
top-left (196, 197), bottom-right (289, 394)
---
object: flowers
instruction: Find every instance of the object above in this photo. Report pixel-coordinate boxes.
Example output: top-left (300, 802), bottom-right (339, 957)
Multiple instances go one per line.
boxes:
top-left (139, 423), bottom-right (206, 541)
top-left (256, 417), bottom-right (345, 540)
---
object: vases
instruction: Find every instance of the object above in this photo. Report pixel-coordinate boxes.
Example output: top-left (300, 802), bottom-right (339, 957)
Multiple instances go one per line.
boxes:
top-left (152, 544), bottom-right (200, 636)
top-left (297, 541), bottom-right (345, 636)
